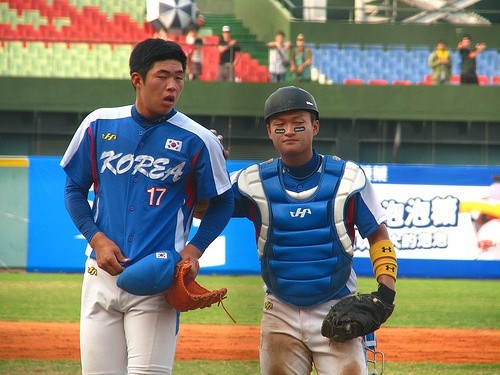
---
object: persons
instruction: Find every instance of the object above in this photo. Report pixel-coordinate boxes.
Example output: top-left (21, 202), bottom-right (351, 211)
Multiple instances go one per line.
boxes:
top-left (425, 38), bottom-right (454, 85)
top-left (184, 38), bottom-right (203, 82)
top-left (457, 33), bottom-right (486, 85)
top-left (59, 39), bottom-right (238, 375)
top-left (194, 85), bottom-right (399, 375)
top-left (288, 34), bottom-right (313, 82)
top-left (216, 25), bottom-right (242, 84)
top-left (264, 31), bottom-right (293, 82)
top-left (154, 13), bottom-right (205, 45)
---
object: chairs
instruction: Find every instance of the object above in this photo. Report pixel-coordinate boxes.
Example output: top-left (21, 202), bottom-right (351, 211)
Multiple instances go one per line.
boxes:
top-left (0, 1), bottom-right (500, 84)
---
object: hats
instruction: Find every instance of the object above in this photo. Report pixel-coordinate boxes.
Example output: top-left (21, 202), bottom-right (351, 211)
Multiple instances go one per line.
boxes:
top-left (296, 34), bottom-right (304, 40)
top-left (222, 26), bottom-right (230, 32)
top-left (117, 249), bottom-right (182, 295)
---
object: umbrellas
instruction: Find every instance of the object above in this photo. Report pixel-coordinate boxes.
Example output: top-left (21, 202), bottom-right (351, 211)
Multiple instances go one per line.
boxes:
top-left (145, 0), bottom-right (202, 34)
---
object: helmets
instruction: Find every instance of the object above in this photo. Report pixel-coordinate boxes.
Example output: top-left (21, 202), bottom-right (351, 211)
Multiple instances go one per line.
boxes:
top-left (264, 86), bottom-right (319, 121)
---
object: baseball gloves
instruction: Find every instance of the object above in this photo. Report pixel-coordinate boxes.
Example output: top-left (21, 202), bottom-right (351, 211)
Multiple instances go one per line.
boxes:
top-left (164, 258), bottom-right (227, 312)
top-left (321, 293), bottom-right (395, 342)
